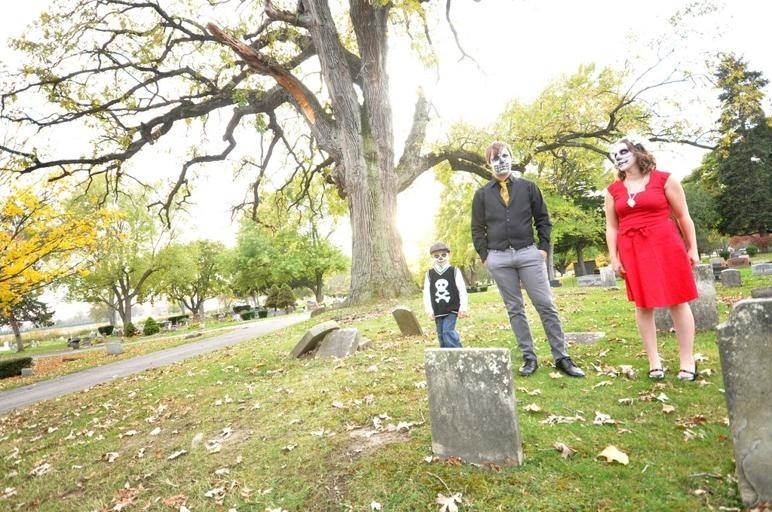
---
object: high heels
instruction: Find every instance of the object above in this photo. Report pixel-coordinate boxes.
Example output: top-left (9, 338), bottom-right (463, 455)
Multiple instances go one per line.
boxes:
top-left (648, 365), bottom-right (665, 380)
top-left (679, 362), bottom-right (698, 381)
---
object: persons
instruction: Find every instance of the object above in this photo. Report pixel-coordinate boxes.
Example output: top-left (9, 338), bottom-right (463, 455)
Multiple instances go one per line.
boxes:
top-left (605, 135), bottom-right (701, 381)
top-left (471, 139), bottom-right (586, 378)
top-left (423, 242), bottom-right (469, 347)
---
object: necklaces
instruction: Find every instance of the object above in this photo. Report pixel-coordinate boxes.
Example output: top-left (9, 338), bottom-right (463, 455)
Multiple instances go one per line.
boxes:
top-left (626, 175), bottom-right (646, 208)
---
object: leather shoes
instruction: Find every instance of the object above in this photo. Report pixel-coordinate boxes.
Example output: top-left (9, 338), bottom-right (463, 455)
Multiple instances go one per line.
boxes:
top-left (555, 356), bottom-right (585, 377)
top-left (518, 359), bottom-right (537, 376)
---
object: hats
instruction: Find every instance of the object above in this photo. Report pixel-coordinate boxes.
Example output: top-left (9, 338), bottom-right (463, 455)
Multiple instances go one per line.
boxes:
top-left (430, 242), bottom-right (450, 255)
